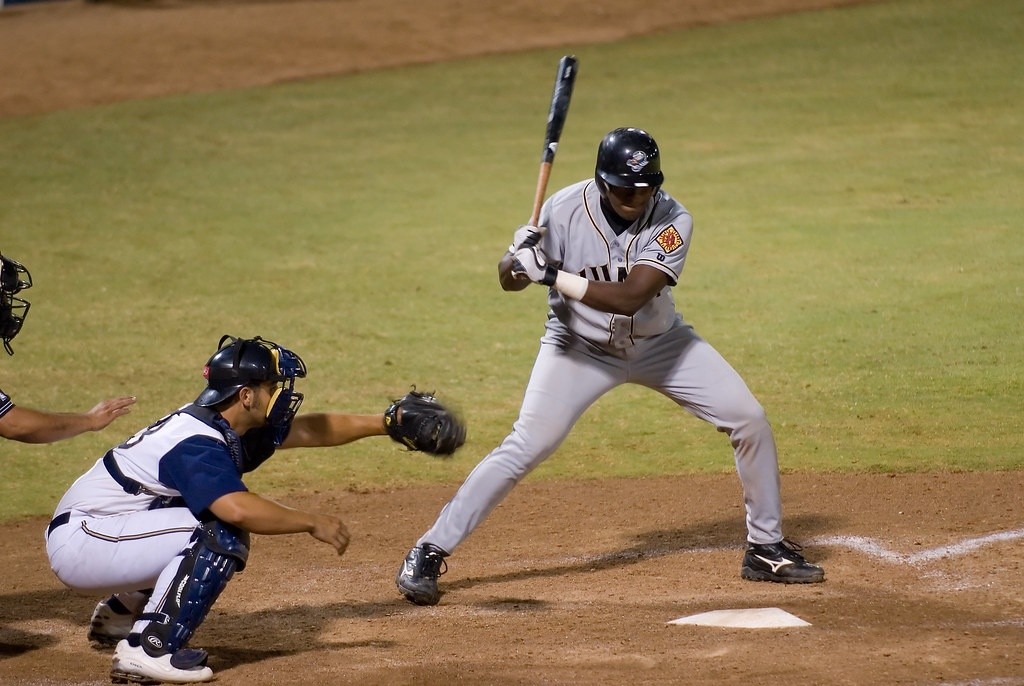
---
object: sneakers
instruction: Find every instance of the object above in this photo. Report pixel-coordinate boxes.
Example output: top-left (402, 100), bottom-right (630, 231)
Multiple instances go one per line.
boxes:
top-left (740, 539), bottom-right (825, 584)
top-left (396, 545), bottom-right (448, 605)
top-left (110, 640), bottom-right (214, 685)
top-left (88, 600), bottom-right (140, 640)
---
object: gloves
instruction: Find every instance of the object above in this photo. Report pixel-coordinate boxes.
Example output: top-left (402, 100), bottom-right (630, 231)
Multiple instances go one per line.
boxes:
top-left (510, 246), bottom-right (589, 301)
top-left (507, 225), bottom-right (547, 253)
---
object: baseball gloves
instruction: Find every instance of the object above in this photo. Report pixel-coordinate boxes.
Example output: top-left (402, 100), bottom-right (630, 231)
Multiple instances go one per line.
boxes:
top-left (383, 388), bottom-right (468, 460)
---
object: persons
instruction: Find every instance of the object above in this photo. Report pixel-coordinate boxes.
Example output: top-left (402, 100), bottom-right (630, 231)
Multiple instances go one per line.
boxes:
top-left (45, 335), bottom-right (404, 683)
top-left (0, 255), bottom-right (137, 443)
top-left (395, 127), bottom-right (825, 608)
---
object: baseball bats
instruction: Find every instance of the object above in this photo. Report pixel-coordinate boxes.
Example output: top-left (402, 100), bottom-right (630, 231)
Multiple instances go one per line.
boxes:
top-left (510, 54), bottom-right (581, 284)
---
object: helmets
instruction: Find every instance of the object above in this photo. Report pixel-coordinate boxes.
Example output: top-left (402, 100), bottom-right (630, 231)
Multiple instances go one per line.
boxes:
top-left (595, 127), bottom-right (665, 187)
top-left (194, 342), bottom-right (274, 408)
